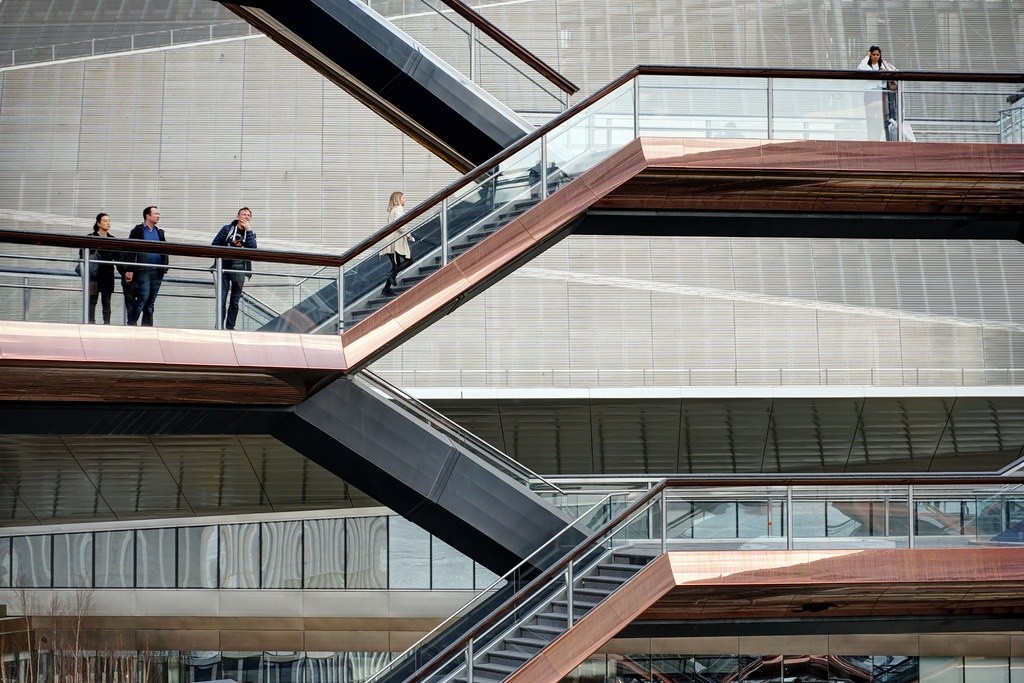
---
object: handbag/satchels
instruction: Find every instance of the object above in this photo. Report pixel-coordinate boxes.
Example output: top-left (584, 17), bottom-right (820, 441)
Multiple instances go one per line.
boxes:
top-left (75, 251), bottom-right (99, 274)
top-left (887, 118), bottom-right (917, 143)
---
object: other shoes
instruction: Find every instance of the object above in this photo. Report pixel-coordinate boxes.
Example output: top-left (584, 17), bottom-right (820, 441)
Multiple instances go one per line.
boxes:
top-left (386, 271), bottom-right (398, 286)
top-left (380, 288), bottom-right (397, 297)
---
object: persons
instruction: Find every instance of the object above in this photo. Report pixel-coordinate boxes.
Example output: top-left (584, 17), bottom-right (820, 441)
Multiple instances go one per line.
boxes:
top-left (125, 206), bottom-right (169, 327)
top-left (379, 191), bottom-right (415, 296)
top-left (857, 46), bottom-right (899, 142)
top-left (212, 207), bottom-right (257, 331)
top-left (79, 213), bottom-right (131, 324)
